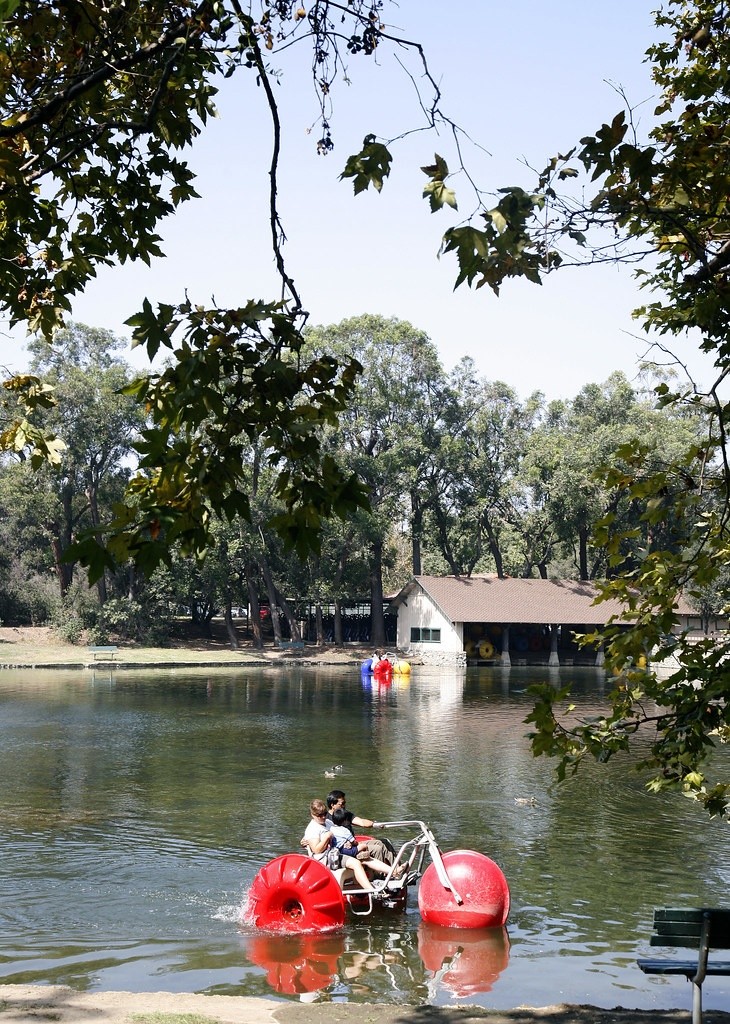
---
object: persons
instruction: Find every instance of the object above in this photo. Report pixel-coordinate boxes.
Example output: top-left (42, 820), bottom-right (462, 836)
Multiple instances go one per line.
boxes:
top-left (330, 809), bottom-right (370, 859)
top-left (304, 798), bottom-right (409, 901)
top-left (300, 790), bottom-right (401, 877)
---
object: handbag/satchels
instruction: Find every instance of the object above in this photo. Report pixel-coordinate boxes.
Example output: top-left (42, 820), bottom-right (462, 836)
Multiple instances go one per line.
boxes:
top-left (327, 847), bottom-right (340, 870)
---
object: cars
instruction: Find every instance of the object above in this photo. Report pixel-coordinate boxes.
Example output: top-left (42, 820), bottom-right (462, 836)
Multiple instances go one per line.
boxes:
top-left (258, 606), bottom-right (271, 620)
top-left (231, 607), bottom-right (247, 618)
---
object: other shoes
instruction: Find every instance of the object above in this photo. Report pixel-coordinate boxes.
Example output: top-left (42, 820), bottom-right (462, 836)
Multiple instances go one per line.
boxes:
top-left (356, 851), bottom-right (370, 859)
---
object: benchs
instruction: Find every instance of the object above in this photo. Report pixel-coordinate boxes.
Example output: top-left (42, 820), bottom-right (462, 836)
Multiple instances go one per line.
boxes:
top-left (278, 642), bottom-right (305, 657)
top-left (636, 908), bottom-right (730, 1024)
top-left (87, 646), bottom-right (119, 661)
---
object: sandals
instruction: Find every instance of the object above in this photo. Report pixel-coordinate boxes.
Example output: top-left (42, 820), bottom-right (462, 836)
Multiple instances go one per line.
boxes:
top-left (393, 861), bottom-right (409, 880)
top-left (372, 893), bottom-right (391, 898)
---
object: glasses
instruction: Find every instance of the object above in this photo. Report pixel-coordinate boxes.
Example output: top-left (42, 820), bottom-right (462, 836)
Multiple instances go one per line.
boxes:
top-left (336, 801), bottom-right (346, 805)
top-left (319, 811), bottom-right (327, 816)
